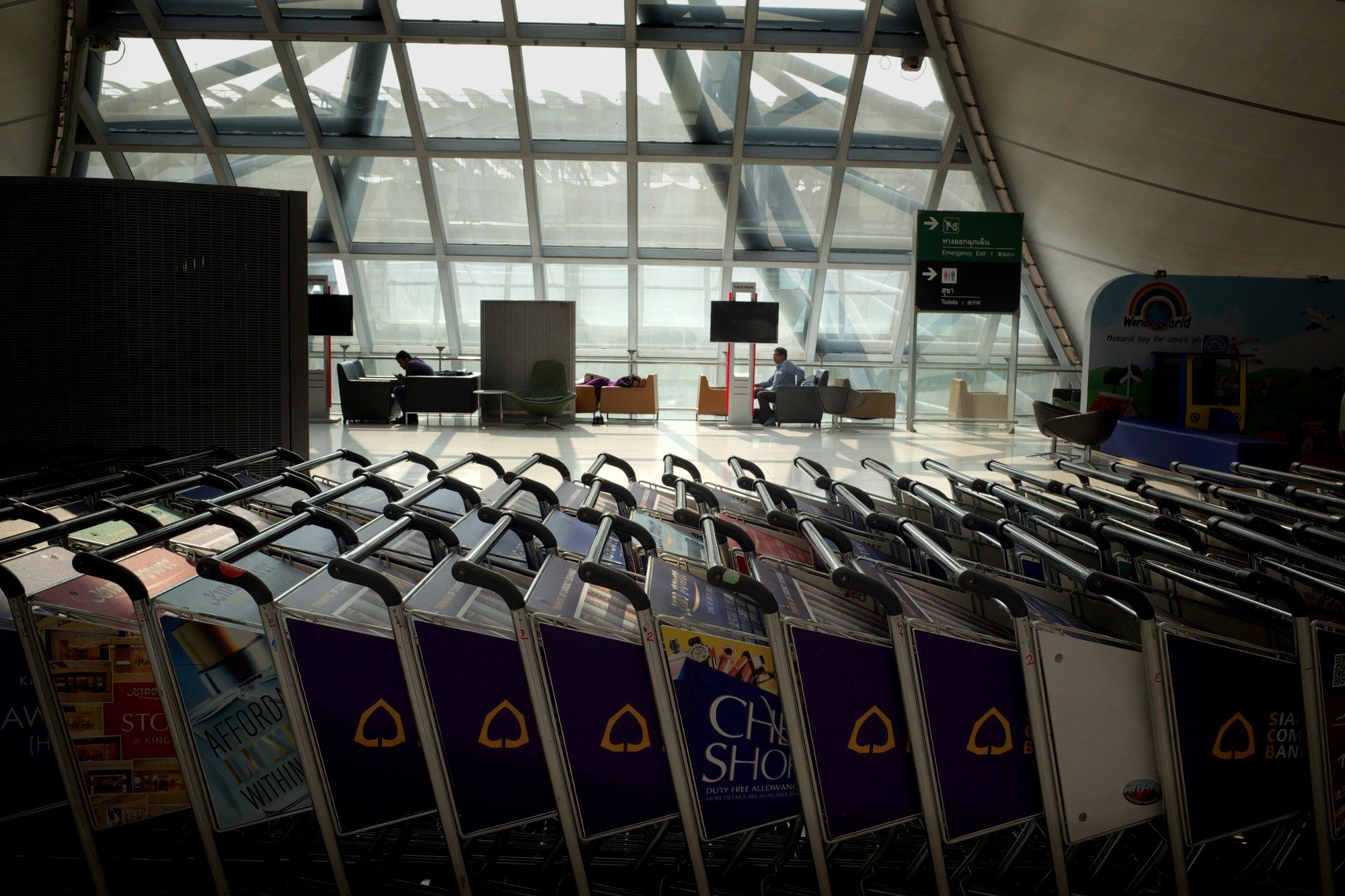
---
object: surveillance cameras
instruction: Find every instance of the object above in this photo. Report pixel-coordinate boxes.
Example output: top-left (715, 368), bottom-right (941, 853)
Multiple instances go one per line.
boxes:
top-left (901, 54), bottom-right (924, 71)
top-left (89, 44), bottom-right (108, 52)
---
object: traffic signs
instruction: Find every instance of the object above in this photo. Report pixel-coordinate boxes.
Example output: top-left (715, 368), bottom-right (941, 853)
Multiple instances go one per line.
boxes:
top-left (914, 206), bottom-right (1025, 315)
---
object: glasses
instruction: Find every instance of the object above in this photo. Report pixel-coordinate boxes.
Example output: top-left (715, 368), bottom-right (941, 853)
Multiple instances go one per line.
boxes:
top-left (773, 353), bottom-right (779, 356)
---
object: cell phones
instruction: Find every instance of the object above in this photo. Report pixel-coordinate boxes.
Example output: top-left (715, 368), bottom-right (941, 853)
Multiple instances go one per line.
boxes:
top-left (392, 373), bottom-right (398, 377)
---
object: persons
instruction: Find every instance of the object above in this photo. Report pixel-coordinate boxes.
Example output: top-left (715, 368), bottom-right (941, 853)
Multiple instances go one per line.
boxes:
top-left (752, 347), bottom-right (805, 426)
top-left (391, 350), bottom-right (437, 424)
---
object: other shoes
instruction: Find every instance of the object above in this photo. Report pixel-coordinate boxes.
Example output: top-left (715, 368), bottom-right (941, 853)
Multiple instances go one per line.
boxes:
top-left (753, 418), bottom-right (767, 424)
top-left (391, 416), bottom-right (418, 426)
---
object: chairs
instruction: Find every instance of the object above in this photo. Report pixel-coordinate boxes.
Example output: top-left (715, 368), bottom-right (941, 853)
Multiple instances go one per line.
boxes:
top-left (507, 360), bottom-right (577, 430)
top-left (1027, 388), bottom-right (1120, 470)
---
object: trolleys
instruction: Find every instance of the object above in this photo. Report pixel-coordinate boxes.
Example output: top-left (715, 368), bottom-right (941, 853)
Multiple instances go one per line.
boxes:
top-left (0, 449), bottom-right (1345, 896)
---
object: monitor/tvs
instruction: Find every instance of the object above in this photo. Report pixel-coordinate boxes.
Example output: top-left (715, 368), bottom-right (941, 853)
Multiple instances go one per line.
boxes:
top-left (710, 301), bottom-right (779, 344)
top-left (308, 294), bottom-right (353, 336)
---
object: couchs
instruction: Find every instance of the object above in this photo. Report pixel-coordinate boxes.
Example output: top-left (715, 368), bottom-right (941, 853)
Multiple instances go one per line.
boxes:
top-left (575, 385), bottom-right (597, 417)
top-left (773, 368), bottom-right (829, 428)
top-left (600, 374), bottom-right (659, 421)
top-left (695, 374), bottom-right (727, 420)
top-left (832, 377), bottom-right (896, 427)
top-left (947, 378), bottom-right (1008, 429)
top-left (337, 361), bottom-right (480, 428)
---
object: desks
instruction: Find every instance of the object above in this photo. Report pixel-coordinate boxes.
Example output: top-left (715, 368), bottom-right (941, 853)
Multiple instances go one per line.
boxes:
top-left (473, 390), bottom-right (508, 428)
